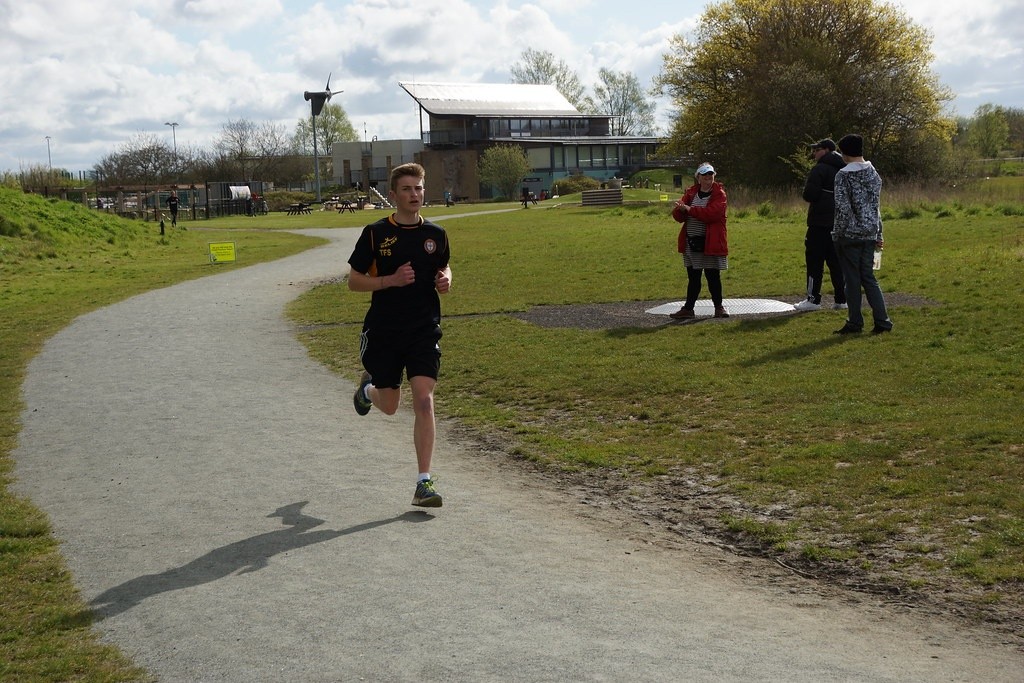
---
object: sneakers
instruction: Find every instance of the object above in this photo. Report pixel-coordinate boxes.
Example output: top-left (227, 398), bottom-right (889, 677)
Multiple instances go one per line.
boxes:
top-left (833, 323), bottom-right (862, 334)
top-left (871, 324), bottom-right (891, 332)
top-left (830, 303), bottom-right (848, 309)
top-left (411, 475), bottom-right (442, 507)
top-left (715, 306), bottom-right (729, 317)
top-left (794, 299), bottom-right (821, 310)
top-left (353, 370), bottom-right (373, 416)
top-left (670, 306), bottom-right (695, 318)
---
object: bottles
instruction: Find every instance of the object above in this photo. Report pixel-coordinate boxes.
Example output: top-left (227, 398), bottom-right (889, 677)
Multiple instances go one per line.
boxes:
top-left (872, 249), bottom-right (882, 270)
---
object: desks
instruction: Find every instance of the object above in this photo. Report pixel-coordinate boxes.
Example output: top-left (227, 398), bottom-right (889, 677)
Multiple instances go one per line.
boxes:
top-left (340, 201), bottom-right (355, 214)
top-left (286, 204), bottom-right (310, 216)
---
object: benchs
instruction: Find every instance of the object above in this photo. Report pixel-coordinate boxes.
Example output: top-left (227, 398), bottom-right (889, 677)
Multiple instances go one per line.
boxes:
top-left (284, 206), bottom-right (359, 214)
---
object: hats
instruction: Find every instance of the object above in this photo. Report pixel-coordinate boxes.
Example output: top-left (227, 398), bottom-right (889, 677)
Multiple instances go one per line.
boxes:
top-left (810, 138), bottom-right (836, 152)
top-left (695, 164), bottom-right (716, 179)
top-left (837, 134), bottom-right (863, 157)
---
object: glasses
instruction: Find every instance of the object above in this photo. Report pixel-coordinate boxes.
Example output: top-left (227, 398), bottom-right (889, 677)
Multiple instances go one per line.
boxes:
top-left (816, 148), bottom-right (825, 151)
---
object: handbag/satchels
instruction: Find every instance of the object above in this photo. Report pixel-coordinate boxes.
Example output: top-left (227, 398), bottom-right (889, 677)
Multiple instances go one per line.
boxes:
top-left (687, 235), bottom-right (705, 253)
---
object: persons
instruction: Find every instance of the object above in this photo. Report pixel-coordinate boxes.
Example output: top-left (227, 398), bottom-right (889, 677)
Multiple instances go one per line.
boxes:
top-left (668, 161), bottom-right (731, 321)
top-left (831, 134), bottom-right (894, 336)
top-left (166, 190), bottom-right (179, 228)
top-left (351, 162), bottom-right (451, 508)
top-left (793, 138), bottom-right (850, 312)
